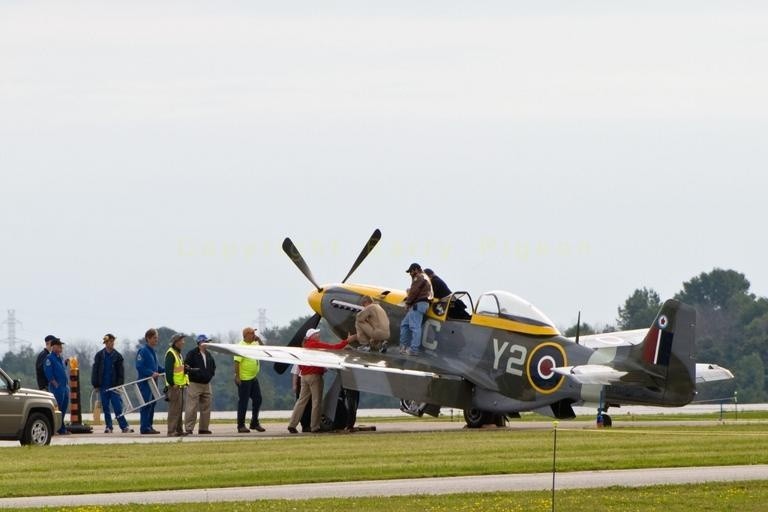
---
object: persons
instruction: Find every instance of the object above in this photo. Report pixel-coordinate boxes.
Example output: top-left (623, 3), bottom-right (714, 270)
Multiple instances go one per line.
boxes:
top-left (287, 328), bottom-right (357, 433)
top-left (424, 267), bottom-right (471, 320)
top-left (291, 335), bottom-right (312, 432)
top-left (184, 334), bottom-right (217, 434)
top-left (233, 326), bottom-right (266, 433)
top-left (90, 333), bottom-right (135, 433)
top-left (355, 293), bottom-right (391, 353)
top-left (398, 262), bottom-right (435, 356)
top-left (43, 337), bottom-right (74, 435)
top-left (163, 333), bottom-right (191, 436)
top-left (35, 334), bottom-right (57, 392)
top-left (135, 327), bottom-right (166, 433)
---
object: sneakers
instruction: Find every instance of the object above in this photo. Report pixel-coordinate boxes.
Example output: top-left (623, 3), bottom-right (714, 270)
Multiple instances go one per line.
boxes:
top-left (122, 426), bottom-right (134, 432)
top-left (400, 345), bottom-right (406, 355)
top-left (168, 431), bottom-right (180, 436)
top-left (379, 339), bottom-right (388, 353)
top-left (60, 431), bottom-right (71, 435)
top-left (406, 348), bottom-right (418, 356)
top-left (199, 431), bottom-right (211, 434)
top-left (176, 431), bottom-right (188, 436)
top-left (105, 426), bottom-right (112, 433)
top-left (186, 430), bottom-right (192, 434)
top-left (311, 427), bottom-right (323, 433)
top-left (288, 426), bottom-right (298, 433)
top-left (357, 345), bottom-right (371, 351)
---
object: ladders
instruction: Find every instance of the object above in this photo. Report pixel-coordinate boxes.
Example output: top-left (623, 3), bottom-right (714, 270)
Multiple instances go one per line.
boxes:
top-left (106, 373), bottom-right (168, 419)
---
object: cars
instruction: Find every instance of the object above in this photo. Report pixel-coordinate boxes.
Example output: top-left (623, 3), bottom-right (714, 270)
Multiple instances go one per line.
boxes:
top-left (0, 367), bottom-right (62, 448)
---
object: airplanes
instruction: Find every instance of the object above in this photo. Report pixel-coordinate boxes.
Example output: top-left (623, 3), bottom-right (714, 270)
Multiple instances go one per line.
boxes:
top-left (198, 227), bottom-right (734, 433)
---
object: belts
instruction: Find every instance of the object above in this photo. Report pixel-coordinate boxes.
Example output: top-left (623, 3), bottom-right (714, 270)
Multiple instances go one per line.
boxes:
top-left (416, 299), bottom-right (426, 302)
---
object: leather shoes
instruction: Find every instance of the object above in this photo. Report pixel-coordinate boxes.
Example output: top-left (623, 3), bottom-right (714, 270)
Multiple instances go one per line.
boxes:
top-left (238, 427), bottom-right (250, 432)
top-left (141, 429), bottom-right (160, 434)
top-left (250, 424), bottom-right (265, 431)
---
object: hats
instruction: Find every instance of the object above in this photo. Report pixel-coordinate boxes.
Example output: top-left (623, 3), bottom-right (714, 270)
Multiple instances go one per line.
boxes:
top-left (196, 335), bottom-right (212, 343)
top-left (306, 328), bottom-right (320, 337)
top-left (45, 336), bottom-right (60, 341)
top-left (406, 263), bottom-right (421, 273)
top-left (168, 333), bottom-right (186, 347)
top-left (51, 340), bottom-right (64, 346)
top-left (103, 334), bottom-right (115, 344)
top-left (243, 327), bottom-right (257, 336)
